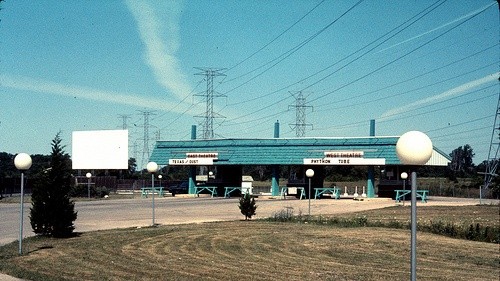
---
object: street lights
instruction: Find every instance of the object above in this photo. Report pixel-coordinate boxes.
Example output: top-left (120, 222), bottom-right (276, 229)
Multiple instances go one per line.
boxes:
top-left (396, 131), bottom-right (433, 281)
top-left (86, 172), bottom-right (92, 199)
top-left (306, 169), bottom-right (315, 216)
top-left (147, 161), bottom-right (163, 225)
top-left (14, 152), bottom-right (33, 256)
